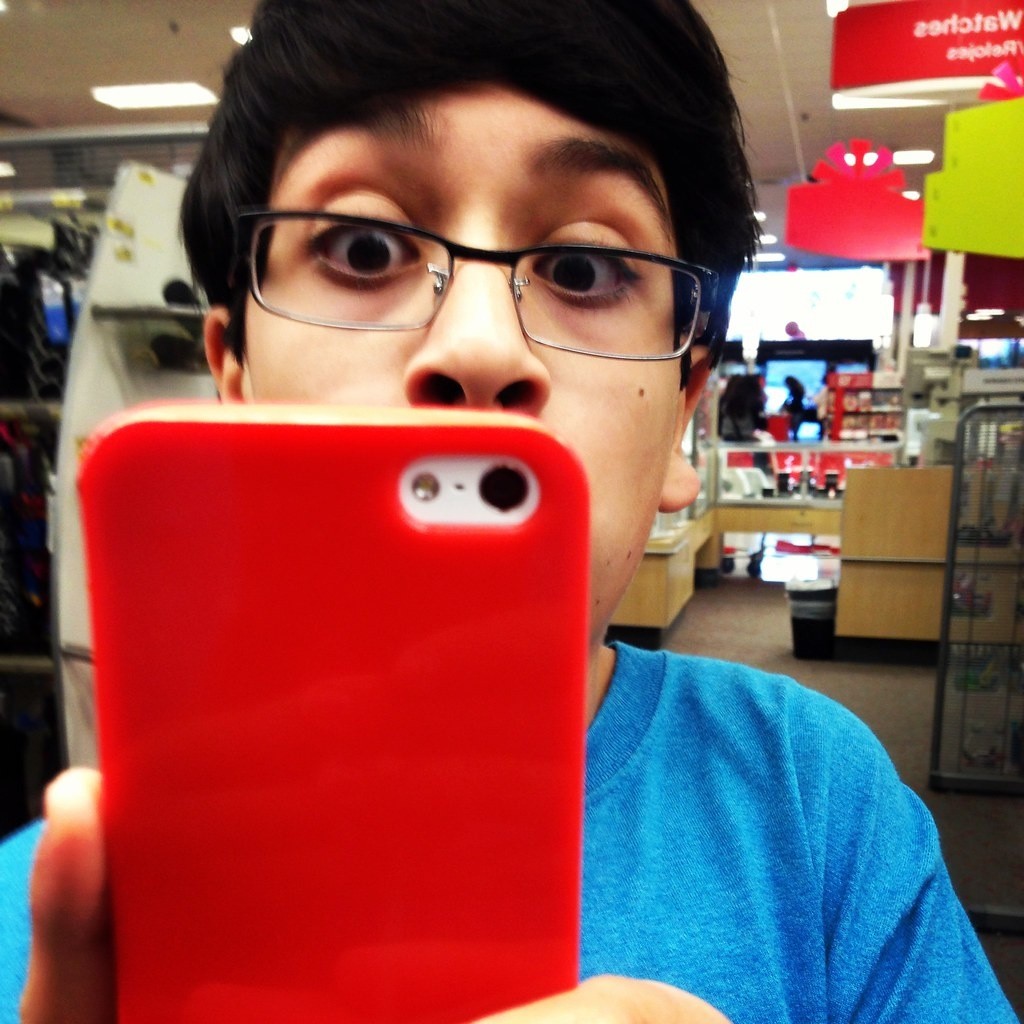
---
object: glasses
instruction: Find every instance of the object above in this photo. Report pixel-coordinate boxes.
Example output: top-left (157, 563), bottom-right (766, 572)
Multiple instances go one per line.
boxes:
top-left (237, 210), bottom-right (719, 362)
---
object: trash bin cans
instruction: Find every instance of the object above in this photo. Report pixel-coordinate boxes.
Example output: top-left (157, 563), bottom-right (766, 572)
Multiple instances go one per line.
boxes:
top-left (785, 578), bottom-right (840, 659)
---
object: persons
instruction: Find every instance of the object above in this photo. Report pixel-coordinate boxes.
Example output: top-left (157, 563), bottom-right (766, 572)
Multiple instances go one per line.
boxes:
top-left (782, 375), bottom-right (805, 440)
top-left (1, 0), bottom-right (1021, 1023)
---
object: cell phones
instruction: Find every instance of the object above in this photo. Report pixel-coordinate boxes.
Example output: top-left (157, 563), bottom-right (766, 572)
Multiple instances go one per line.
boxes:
top-left (76, 403), bottom-right (590, 1024)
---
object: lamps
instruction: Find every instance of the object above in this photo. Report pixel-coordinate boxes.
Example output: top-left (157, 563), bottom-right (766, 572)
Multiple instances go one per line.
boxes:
top-left (912, 257), bottom-right (934, 348)
